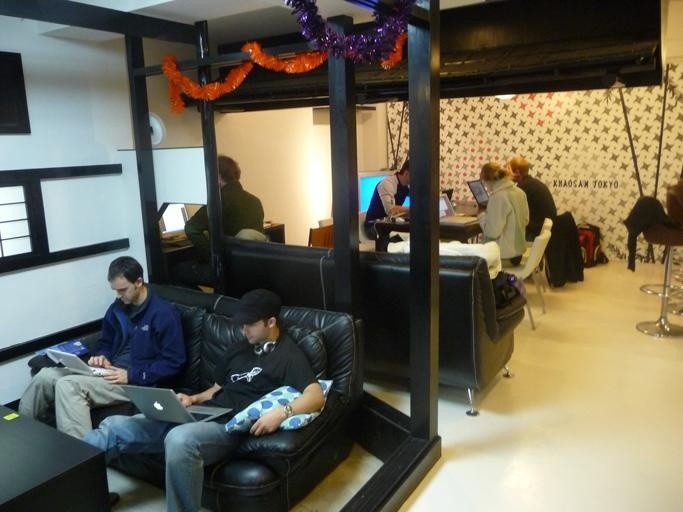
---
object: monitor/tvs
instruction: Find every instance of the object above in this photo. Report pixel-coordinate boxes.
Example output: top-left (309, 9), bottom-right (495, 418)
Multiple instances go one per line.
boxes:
top-left (467, 179), bottom-right (489, 207)
top-left (442, 188), bottom-right (453, 200)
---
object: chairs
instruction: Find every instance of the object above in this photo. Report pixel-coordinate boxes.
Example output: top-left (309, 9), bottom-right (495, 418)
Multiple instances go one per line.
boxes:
top-left (636, 185), bottom-right (683, 340)
top-left (502, 216), bottom-right (554, 332)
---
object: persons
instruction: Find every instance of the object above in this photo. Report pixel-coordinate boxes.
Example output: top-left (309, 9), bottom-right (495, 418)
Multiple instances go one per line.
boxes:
top-left (17, 255), bottom-right (187, 442)
top-left (183, 155), bottom-right (265, 288)
top-left (501, 157), bottom-right (557, 244)
top-left (80, 288), bottom-right (325, 512)
top-left (362, 159), bottom-right (410, 244)
top-left (474, 162), bottom-right (530, 268)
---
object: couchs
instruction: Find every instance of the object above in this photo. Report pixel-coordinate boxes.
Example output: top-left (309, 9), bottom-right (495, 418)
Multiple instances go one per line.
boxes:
top-left (221, 232), bottom-right (526, 417)
top-left (33, 280), bottom-right (367, 512)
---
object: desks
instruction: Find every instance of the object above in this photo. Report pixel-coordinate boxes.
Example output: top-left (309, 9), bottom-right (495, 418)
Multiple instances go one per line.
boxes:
top-left (366, 201), bottom-right (487, 251)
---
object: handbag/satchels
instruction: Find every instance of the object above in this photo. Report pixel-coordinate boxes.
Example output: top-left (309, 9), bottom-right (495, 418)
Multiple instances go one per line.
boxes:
top-left (577, 223), bottom-right (602, 267)
top-left (492, 272), bottom-right (519, 308)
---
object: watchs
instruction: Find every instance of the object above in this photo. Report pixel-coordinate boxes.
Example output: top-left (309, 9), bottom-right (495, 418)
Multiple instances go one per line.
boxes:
top-left (283, 404), bottom-right (295, 418)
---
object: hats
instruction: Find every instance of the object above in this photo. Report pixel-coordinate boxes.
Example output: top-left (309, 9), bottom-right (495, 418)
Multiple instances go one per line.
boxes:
top-left (232, 289), bottom-right (280, 324)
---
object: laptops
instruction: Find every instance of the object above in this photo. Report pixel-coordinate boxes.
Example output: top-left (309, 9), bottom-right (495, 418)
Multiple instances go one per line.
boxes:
top-left (120, 383), bottom-right (234, 424)
top-left (48, 348), bottom-right (114, 376)
top-left (439, 193), bottom-right (479, 225)
top-left (157, 203), bottom-right (200, 245)
